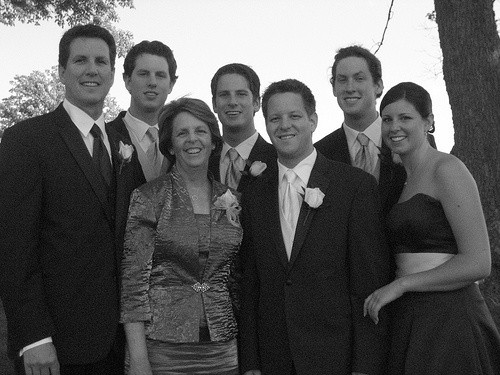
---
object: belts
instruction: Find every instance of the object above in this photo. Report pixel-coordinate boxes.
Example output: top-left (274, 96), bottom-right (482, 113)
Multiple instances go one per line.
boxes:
top-left (90, 123), bottom-right (112, 186)
top-left (354, 133), bottom-right (373, 176)
top-left (146, 126), bottom-right (164, 169)
top-left (225, 148), bottom-right (242, 192)
top-left (281, 170), bottom-right (300, 233)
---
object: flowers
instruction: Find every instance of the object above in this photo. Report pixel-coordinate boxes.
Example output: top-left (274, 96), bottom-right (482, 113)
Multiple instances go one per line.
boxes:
top-left (239, 159), bottom-right (267, 176)
top-left (118, 141), bottom-right (135, 175)
top-left (212, 189), bottom-right (243, 228)
top-left (298, 186), bottom-right (326, 226)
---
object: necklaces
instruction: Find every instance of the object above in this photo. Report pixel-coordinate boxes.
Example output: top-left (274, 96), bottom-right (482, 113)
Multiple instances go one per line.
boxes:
top-left (187, 184), bottom-right (208, 201)
top-left (403, 166), bottom-right (417, 185)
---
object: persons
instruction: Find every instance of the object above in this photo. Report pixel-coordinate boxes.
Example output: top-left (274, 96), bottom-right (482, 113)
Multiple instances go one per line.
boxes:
top-left (0, 22), bottom-right (115, 375)
top-left (239, 78), bottom-right (387, 375)
top-left (208, 63), bottom-right (276, 193)
top-left (105, 40), bottom-right (179, 188)
top-left (119, 97), bottom-right (244, 375)
top-left (311, 45), bottom-right (436, 214)
top-left (363, 82), bottom-right (500, 375)
